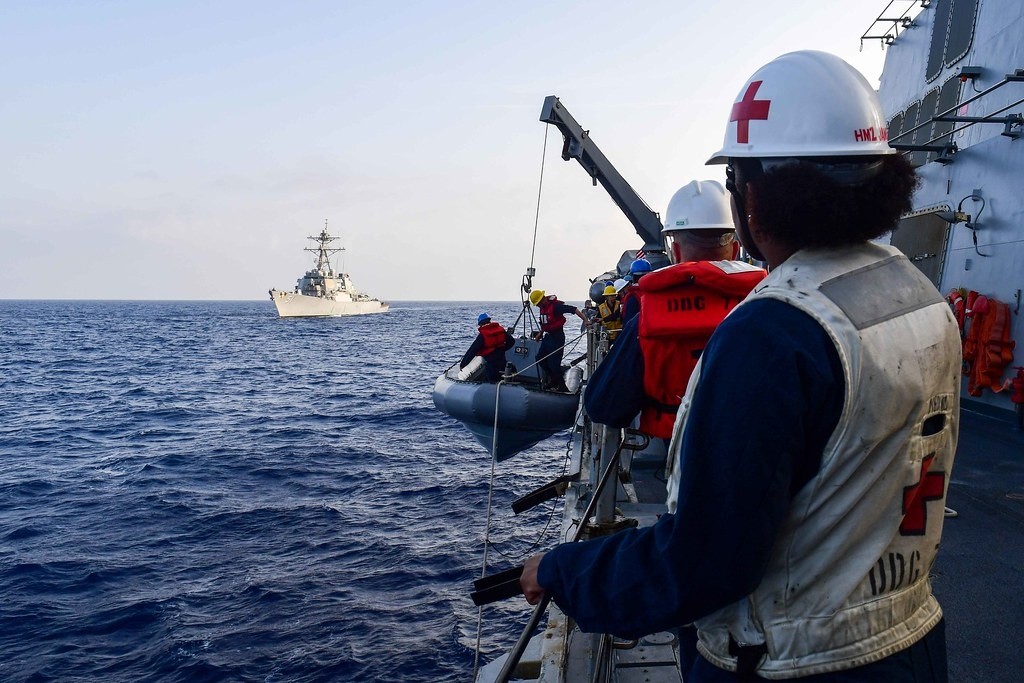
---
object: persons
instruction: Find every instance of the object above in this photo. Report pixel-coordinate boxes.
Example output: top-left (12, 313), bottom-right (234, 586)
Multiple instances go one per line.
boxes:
top-left (530, 289), bottom-right (591, 391)
top-left (460, 312), bottom-right (515, 382)
top-left (580, 258), bottom-right (653, 351)
top-left (521, 46), bottom-right (962, 682)
top-left (580, 180), bottom-right (769, 473)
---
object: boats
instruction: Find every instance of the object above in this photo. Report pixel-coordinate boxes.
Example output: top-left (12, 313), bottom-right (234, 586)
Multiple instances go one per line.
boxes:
top-left (433, 331), bottom-right (584, 463)
top-left (269, 219), bottom-right (390, 316)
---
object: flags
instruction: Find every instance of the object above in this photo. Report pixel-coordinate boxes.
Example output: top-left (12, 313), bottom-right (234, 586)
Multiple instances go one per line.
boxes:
top-left (636, 248), bottom-right (646, 260)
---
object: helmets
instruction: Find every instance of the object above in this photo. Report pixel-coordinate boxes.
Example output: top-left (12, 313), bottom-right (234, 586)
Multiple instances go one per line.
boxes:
top-left (704, 50), bottom-right (898, 165)
top-left (623, 275), bottom-right (633, 280)
top-left (659, 180), bottom-right (736, 232)
top-left (606, 281), bottom-right (614, 286)
top-left (530, 290), bottom-right (545, 305)
top-left (477, 313), bottom-right (491, 325)
top-left (629, 258), bottom-right (653, 272)
top-left (614, 279), bottom-right (628, 291)
top-left (602, 286), bottom-right (618, 295)
